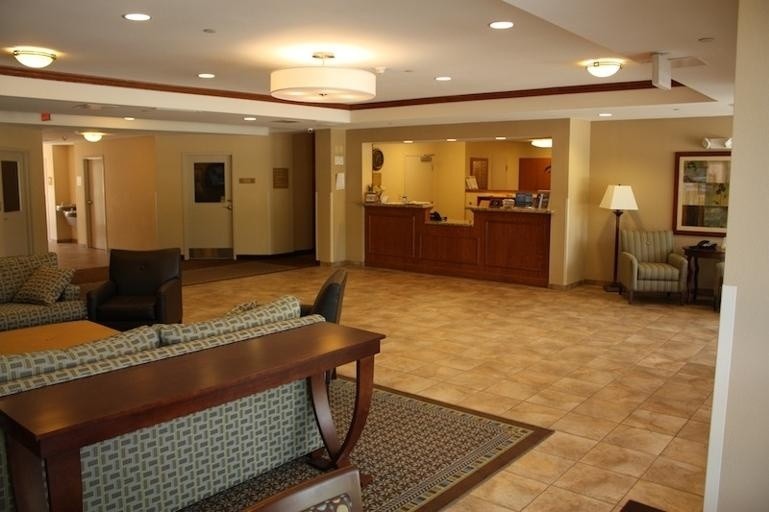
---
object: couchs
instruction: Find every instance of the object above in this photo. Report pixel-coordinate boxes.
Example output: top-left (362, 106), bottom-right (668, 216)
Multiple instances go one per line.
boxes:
top-left (0, 296), bottom-right (327, 512)
top-left (0, 252), bottom-right (88, 331)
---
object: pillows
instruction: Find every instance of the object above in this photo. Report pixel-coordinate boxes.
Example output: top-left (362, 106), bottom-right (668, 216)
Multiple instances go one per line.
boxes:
top-left (13, 264), bottom-right (75, 304)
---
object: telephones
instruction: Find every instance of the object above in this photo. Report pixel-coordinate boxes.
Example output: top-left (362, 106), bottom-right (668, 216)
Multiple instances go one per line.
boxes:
top-left (697, 239), bottom-right (718, 249)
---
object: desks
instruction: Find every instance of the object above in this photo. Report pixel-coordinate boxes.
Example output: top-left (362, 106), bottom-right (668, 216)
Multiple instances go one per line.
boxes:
top-left (1, 321), bottom-right (386, 512)
top-left (684, 245), bottom-right (725, 304)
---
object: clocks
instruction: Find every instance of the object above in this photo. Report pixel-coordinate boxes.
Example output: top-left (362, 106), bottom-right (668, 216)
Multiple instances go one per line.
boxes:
top-left (372, 148), bottom-right (384, 171)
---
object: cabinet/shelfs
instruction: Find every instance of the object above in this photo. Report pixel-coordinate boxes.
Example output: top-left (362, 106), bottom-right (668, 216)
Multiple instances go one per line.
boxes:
top-left (465, 191), bottom-right (517, 225)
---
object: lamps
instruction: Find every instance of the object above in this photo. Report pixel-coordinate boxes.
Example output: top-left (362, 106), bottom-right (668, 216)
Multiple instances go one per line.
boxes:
top-left (78, 132), bottom-right (107, 143)
top-left (269, 51), bottom-right (376, 104)
top-left (587, 61), bottom-right (621, 78)
top-left (599, 184), bottom-right (639, 292)
top-left (12, 49), bottom-right (57, 69)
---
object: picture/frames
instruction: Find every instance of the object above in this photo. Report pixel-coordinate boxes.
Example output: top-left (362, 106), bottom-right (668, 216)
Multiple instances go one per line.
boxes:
top-left (672, 151), bottom-right (731, 238)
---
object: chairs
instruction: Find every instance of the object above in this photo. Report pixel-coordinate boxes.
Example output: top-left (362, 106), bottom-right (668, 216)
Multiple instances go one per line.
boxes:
top-left (301, 269), bottom-right (348, 384)
top-left (86, 248), bottom-right (183, 332)
top-left (239, 465), bottom-right (363, 512)
top-left (619, 229), bottom-right (689, 305)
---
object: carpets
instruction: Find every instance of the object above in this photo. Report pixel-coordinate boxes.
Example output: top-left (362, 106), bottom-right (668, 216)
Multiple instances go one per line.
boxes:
top-left (72, 255), bottom-right (319, 300)
top-left (174, 371), bottom-right (555, 512)
